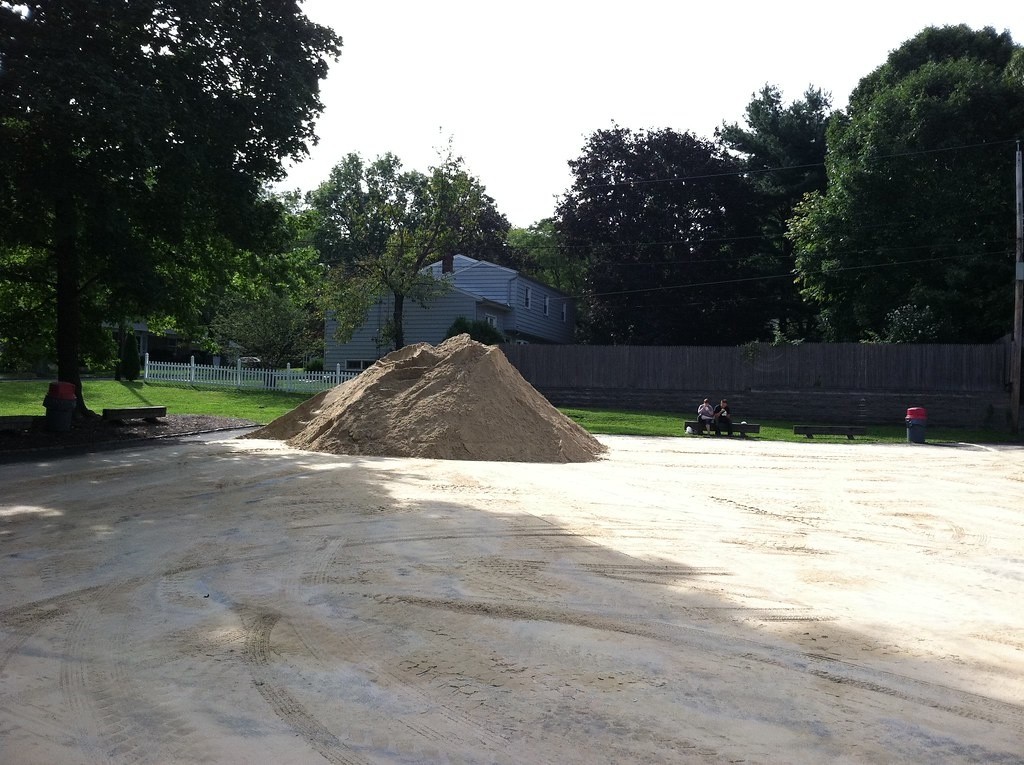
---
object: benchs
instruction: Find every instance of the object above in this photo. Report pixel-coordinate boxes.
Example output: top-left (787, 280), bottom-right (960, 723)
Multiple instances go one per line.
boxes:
top-left (103, 406), bottom-right (167, 424)
top-left (684, 421), bottom-right (760, 438)
top-left (793, 425), bottom-right (864, 440)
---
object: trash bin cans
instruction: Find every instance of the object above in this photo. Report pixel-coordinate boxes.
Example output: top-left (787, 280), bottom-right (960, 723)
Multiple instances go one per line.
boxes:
top-left (905, 407), bottom-right (926, 443)
top-left (45, 381), bottom-right (77, 432)
top-left (263, 370), bottom-right (277, 388)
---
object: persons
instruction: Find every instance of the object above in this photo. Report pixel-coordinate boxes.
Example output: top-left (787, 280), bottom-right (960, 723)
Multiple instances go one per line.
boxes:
top-left (697, 399), bottom-right (714, 437)
top-left (714, 399), bottom-right (734, 437)
